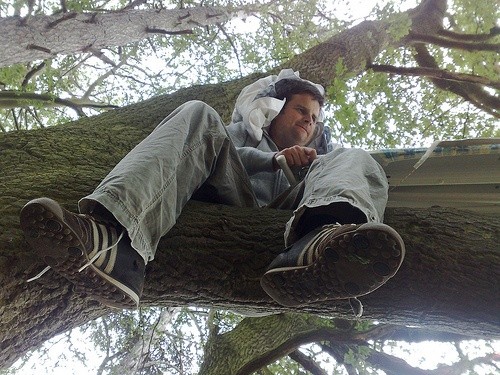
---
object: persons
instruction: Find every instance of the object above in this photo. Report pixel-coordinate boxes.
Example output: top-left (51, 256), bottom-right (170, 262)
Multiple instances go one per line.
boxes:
top-left (19, 69), bottom-right (405, 309)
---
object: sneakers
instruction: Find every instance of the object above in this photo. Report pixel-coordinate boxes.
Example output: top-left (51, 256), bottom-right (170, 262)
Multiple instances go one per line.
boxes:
top-left (20, 198), bottom-right (144, 310)
top-left (260, 223), bottom-right (406, 318)
